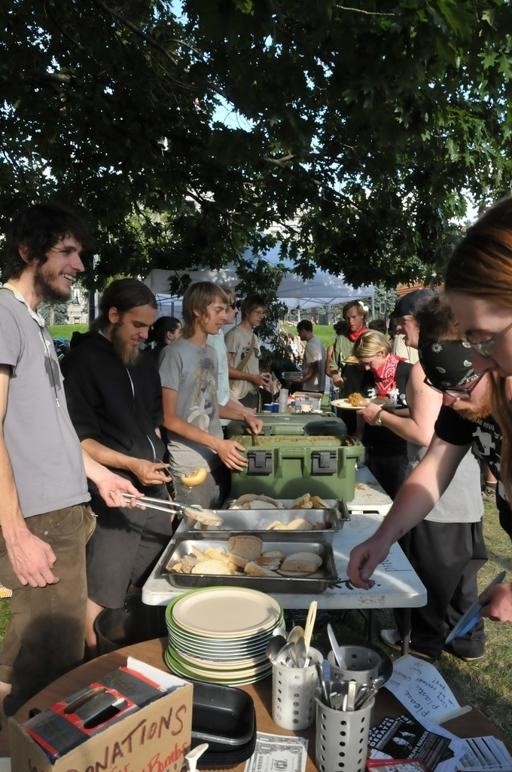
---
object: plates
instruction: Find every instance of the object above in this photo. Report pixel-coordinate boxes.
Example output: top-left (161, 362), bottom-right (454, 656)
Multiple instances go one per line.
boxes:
top-left (445, 570), bottom-right (505, 645)
top-left (330, 396), bottom-right (402, 410)
top-left (165, 586), bottom-right (286, 687)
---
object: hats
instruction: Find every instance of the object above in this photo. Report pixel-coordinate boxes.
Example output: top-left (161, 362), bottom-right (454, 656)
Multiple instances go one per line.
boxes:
top-left (389, 288), bottom-right (435, 319)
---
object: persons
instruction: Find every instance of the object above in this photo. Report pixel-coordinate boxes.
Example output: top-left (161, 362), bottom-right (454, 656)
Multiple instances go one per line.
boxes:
top-left (65, 274), bottom-right (177, 651)
top-left (442, 192), bottom-right (510, 514)
top-left (158, 280), bottom-right (264, 523)
top-left (223, 292), bottom-right (274, 437)
top-left (205, 288), bottom-right (258, 440)
top-left (0, 195), bottom-right (149, 710)
top-left (292, 287), bottom-right (511, 671)
top-left (149, 316), bottom-right (184, 349)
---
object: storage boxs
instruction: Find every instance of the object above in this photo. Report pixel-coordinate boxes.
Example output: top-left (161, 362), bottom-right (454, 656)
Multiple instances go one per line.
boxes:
top-left (7, 657), bottom-right (195, 772)
top-left (225, 413), bottom-right (366, 503)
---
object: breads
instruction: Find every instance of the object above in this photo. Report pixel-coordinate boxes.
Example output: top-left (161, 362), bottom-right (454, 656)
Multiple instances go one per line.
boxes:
top-left (168, 494), bottom-right (334, 579)
top-left (348, 392), bottom-right (363, 408)
top-left (181, 466), bottom-right (207, 488)
top-left (344, 354), bottom-right (359, 362)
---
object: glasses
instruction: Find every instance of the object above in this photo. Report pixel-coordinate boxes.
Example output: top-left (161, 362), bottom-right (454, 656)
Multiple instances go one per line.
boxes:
top-left (460, 323), bottom-right (512, 360)
top-left (424, 367), bottom-right (491, 400)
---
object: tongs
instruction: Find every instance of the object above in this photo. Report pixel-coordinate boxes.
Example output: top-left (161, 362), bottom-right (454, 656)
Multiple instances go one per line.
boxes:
top-left (158, 458), bottom-right (192, 489)
top-left (122, 492), bottom-right (202, 516)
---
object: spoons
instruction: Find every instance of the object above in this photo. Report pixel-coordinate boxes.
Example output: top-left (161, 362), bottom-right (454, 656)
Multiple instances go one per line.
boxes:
top-left (267, 626), bottom-right (307, 667)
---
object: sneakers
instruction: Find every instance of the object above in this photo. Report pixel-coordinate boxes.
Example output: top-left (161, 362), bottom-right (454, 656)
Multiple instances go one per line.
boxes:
top-left (442, 634), bottom-right (484, 662)
top-left (380, 628), bottom-right (441, 660)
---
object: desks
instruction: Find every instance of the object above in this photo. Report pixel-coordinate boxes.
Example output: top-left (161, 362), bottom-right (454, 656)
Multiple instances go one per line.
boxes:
top-left (0, 634), bottom-right (512, 772)
